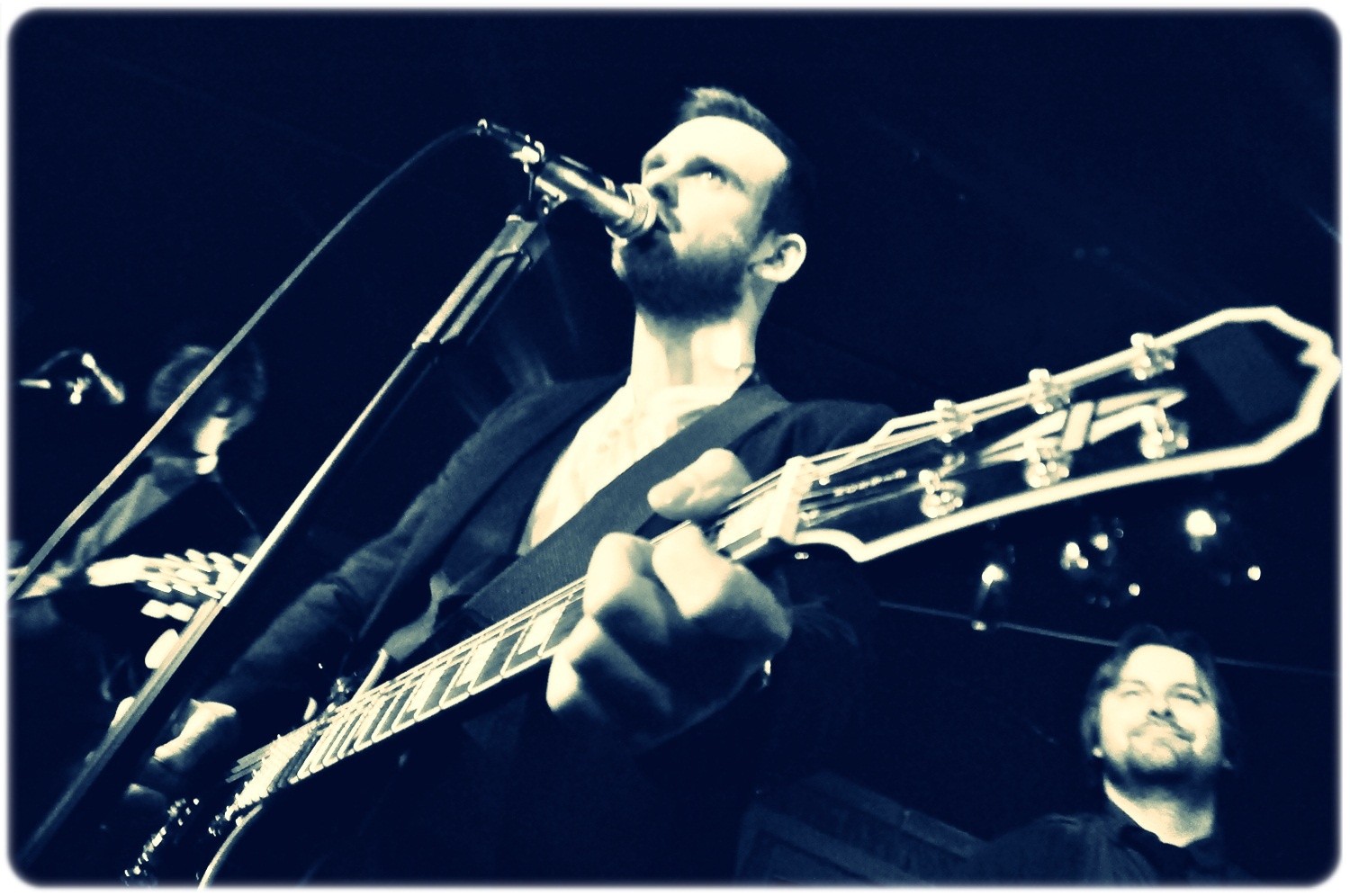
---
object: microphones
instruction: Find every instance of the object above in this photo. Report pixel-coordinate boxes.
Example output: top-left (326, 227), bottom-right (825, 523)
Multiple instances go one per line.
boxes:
top-left (79, 351), bottom-right (125, 403)
top-left (477, 118), bottom-right (662, 242)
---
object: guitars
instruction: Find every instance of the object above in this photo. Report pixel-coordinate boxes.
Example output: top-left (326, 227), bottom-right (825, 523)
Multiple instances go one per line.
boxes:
top-left (121, 306), bottom-right (1343, 887)
top-left (7, 547), bottom-right (250, 602)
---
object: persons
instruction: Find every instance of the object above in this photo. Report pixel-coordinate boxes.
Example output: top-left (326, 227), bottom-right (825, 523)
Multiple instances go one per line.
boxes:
top-left (149, 84), bottom-right (970, 886)
top-left (955, 624), bottom-right (1288, 887)
top-left (10, 331), bottom-right (265, 765)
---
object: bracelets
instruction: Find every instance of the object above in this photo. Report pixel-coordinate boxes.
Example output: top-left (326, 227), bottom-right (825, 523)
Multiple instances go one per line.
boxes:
top-left (751, 650), bottom-right (777, 706)
